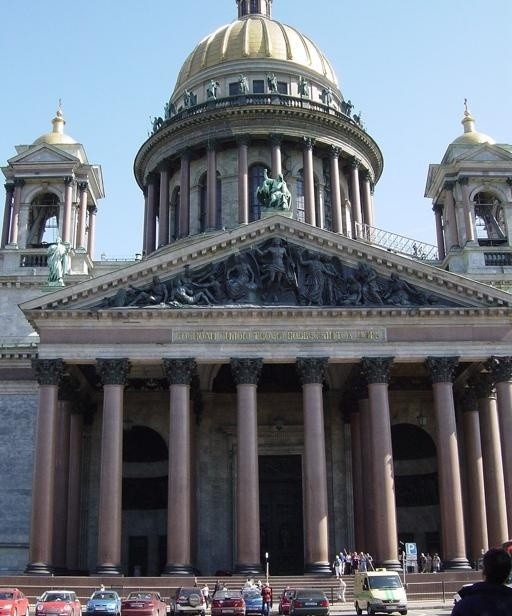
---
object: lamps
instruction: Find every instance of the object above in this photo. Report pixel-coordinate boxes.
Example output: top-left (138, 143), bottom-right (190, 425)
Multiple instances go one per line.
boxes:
top-left (417, 399), bottom-right (428, 427)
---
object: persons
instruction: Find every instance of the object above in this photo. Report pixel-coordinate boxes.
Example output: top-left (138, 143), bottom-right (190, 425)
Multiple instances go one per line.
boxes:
top-left (451, 548), bottom-right (512, 616)
top-left (332, 551), bottom-right (373, 580)
top-left (193, 577), bottom-right (228, 608)
top-left (45, 237), bottom-right (67, 286)
top-left (336, 578), bottom-right (346, 603)
top-left (100, 584), bottom-right (105, 598)
top-left (419, 552), bottom-right (441, 574)
top-left (260, 583), bottom-right (272, 616)
top-left (127, 237), bottom-right (420, 308)
top-left (263, 169), bottom-right (287, 209)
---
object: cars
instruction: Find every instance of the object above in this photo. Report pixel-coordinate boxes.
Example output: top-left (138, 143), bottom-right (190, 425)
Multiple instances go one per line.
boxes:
top-left (242, 589), bottom-right (272, 614)
top-left (210, 590), bottom-right (246, 616)
top-left (86, 591), bottom-right (121, 615)
top-left (0, 587), bottom-right (29, 616)
top-left (35, 591), bottom-right (82, 616)
top-left (121, 591), bottom-right (167, 616)
top-left (279, 585), bottom-right (330, 616)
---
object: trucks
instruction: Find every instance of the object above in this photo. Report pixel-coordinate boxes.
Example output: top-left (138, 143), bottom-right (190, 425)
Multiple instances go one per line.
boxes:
top-left (353, 570), bottom-right (408, 615)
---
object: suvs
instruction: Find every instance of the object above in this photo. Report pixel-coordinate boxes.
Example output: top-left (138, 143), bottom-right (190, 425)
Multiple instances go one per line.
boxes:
top-left (171, 586), bottom-right (206, 616)
